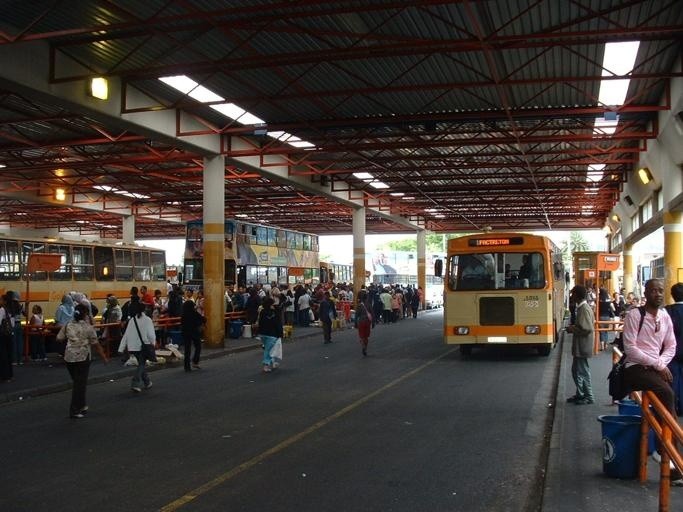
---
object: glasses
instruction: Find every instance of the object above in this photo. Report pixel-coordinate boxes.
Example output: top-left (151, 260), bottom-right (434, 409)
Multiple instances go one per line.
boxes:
top-left (655, 315), bottom-right (660, 332)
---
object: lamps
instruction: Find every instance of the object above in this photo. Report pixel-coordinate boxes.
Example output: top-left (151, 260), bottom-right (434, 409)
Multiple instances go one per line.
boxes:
top-left (85, 72), bottom-right (110, 103)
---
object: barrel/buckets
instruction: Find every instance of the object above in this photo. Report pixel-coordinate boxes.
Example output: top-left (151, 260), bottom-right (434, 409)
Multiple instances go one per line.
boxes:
top-left (168, 330), bottom-right (182, 348)
top-left (597, 414), bottom-right (642, 480)
top-left (242, 325), bottom-right (252, 338)
top-left (229, 320), bottom-right (242, 339)
top-left (284, 325), bottom-right (292, 338)
top-left (615, 398), bottom-right (656, 454)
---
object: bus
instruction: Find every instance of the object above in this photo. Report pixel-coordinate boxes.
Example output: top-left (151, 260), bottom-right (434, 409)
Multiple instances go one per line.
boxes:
top-left (636, 254), bottom-right (664, 301)
top-left (434, 227), bottom-right (570, 355)
top-left (178, 218), bottom-right (322, 294)
top-left (319, 262), bottom-right (371, 290)
top-left (0, 231), bottom-right (171, 326)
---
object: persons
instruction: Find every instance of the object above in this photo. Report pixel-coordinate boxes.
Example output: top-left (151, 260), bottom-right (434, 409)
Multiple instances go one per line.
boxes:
top-left (460, 253), bottom-right (535, 290)
top-left (564, 277), bottom-right (682, 487)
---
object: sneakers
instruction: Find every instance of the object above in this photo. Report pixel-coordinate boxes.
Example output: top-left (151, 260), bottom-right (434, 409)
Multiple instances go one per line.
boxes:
top-left (652, 448), bottom-right (683, 487)
top-left (70, 405), bottom-right (89, 418)
top-left (146, 381), bottom-right (152, 389)
top-left (130, 387), bottom-right (141, 392)
top-left (567, 395), bottom-right (595, 404)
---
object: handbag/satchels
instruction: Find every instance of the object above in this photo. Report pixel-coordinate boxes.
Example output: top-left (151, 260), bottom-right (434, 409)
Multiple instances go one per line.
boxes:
top-left (269, 337), bottom-right (282, 361)
top-left (0, 317), bottom-right (14, 337)
top-left (141, 343), bottom-right (157, 362)
top-left (607, 362), bottom-right (631, 400)
top-left (367, 311), bottom-right (373, 322)
